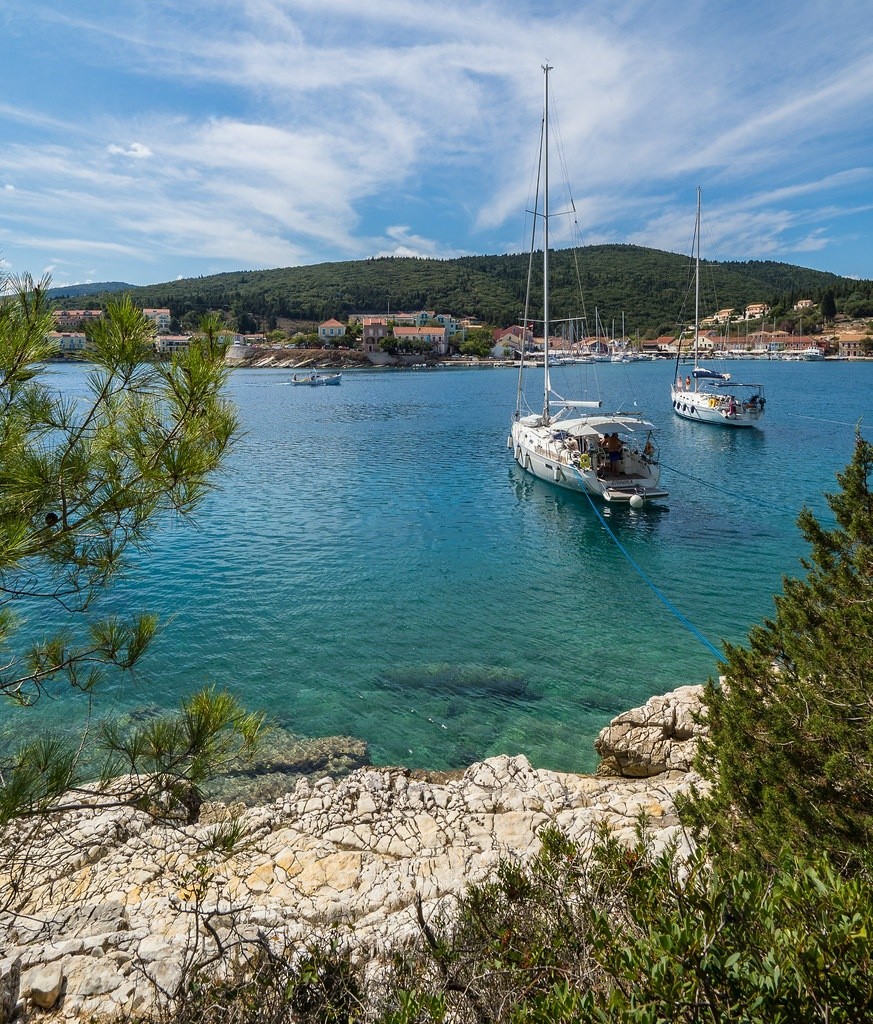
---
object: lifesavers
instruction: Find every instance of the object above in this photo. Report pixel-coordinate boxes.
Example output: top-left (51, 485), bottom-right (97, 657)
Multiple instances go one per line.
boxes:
top-left (708, 399), bottom-right (716, 407)
top-left (579, 454), bottom-right (590, 466)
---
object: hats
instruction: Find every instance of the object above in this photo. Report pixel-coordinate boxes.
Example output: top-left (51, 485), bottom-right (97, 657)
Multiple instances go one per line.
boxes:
top-left (604, 434), bottom-right (609, 438)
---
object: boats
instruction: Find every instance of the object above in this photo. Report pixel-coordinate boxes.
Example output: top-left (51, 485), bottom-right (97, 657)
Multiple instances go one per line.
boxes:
top-left (288, 371), bottom-right (343, 388)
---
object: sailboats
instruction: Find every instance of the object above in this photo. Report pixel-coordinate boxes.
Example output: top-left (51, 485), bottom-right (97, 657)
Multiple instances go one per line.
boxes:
top-left (671, 184), bottom-right (769, 428)
top-left (711, 313), bottom-right (826, 362)
top-left (508, 61), bottom-right (671, 505)
top-left (549, 307), bottom-right (667, 364)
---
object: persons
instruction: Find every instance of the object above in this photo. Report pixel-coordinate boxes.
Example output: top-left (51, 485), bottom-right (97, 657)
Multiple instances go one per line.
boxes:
top-left (641, 441), bottom-right (653, 464)
top-left (748, 394), bottom-right (759, 408)
top-left (726, 396), bottom-right (741, 420)
top-left (599, 433), bottom-right (624, 479)
